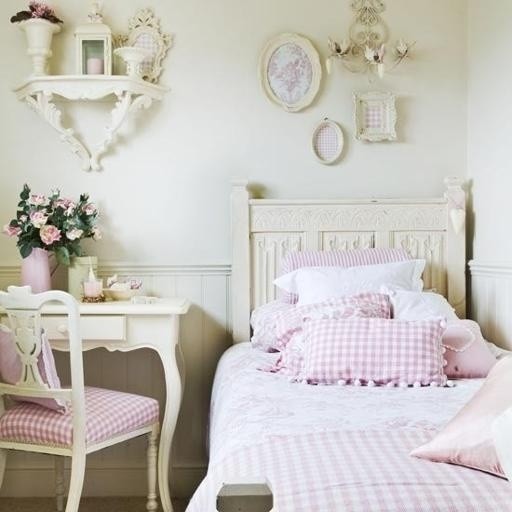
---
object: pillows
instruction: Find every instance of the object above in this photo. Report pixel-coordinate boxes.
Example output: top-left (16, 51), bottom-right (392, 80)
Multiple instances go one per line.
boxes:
top-left (250, 248), bottom-right (503, 386)
top-left (409, 354), bottom-right (512, 481)
top-left (1, 324), bottom-right (68, 415)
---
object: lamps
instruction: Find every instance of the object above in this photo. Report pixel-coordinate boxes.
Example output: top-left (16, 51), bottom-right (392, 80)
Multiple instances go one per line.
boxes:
top-left (325, 0), bottom-right (420, 79)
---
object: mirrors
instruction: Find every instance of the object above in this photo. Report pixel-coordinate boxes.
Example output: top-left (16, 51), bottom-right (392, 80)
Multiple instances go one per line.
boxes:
top-left (133, 30), bottom-right (161, 76)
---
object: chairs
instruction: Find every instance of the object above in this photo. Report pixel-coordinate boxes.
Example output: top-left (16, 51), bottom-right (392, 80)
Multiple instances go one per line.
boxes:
top-left (0, 290), bottom-right (159, 512)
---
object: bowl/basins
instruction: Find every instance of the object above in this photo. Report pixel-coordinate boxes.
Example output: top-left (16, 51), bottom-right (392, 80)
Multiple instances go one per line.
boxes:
top-left (102, 288), bottom-right (140, 301)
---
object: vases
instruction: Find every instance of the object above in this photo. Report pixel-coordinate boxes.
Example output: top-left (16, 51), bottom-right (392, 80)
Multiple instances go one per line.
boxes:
top-left (18, 18), bottom-right (61, 76)
top-left (20, 247), bottom-right (52, 293)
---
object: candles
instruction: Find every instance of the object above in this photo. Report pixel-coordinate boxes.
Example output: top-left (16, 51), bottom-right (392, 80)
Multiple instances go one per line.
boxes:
top-left (84, 268), bottom-right (101, 296)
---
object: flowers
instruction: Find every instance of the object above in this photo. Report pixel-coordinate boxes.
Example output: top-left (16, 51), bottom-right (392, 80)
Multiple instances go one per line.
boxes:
top-left (1, 183), bottom-right (102, 265)
top-left (10, 1), bottom-right (63, 23)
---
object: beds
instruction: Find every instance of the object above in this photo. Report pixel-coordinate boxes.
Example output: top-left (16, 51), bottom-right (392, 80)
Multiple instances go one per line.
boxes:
top-left (185, 178), bottom-right (512, 512)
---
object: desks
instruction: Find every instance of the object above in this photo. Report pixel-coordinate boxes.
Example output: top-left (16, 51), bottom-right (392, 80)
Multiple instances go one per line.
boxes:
top-left (0, 298), bottom-right (191, 512)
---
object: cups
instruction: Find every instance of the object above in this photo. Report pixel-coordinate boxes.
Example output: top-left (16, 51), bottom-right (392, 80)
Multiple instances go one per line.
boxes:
top-left (19, 245), bottom-right (61, 293)
top-left (86, 58), bottom-right (103, 74)
top-left (80, 278), bottom-right (104, 298)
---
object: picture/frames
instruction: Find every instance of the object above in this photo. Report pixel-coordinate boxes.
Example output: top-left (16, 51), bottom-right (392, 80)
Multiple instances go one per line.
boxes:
top-left (352, 89), bottom-right (399, 142)
top-left (259, 33), bottom-right (322, 111)
top-left (311, 121), bottom-right (344, 165)
